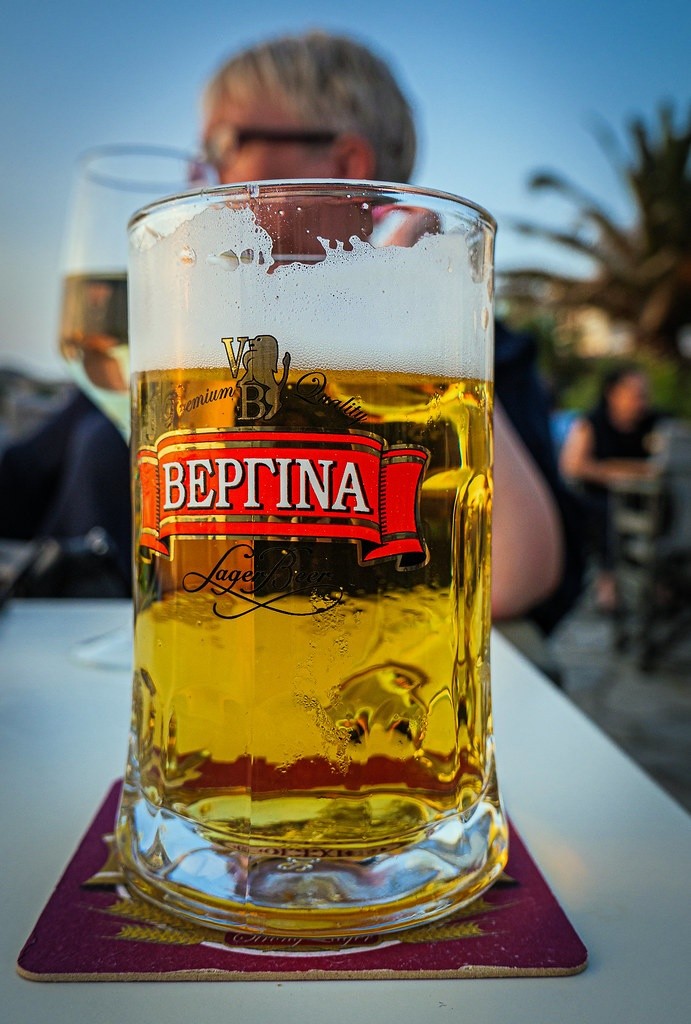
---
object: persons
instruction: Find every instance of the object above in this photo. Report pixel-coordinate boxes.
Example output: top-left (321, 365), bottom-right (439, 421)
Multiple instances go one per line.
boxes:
top-left (557, 367), bottom-right (675, 614)
top-left (199, 36), bottom-right (564, 629)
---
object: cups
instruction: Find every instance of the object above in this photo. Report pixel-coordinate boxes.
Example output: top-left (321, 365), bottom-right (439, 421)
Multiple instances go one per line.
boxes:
top-left (55, 142), bottom-right (218, 359)
top-left (127, 178), bottom-right (509, 938)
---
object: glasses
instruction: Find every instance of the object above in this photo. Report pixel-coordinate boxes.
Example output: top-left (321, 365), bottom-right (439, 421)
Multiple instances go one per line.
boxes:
top-left (189, 123), bottom-right (341, 182)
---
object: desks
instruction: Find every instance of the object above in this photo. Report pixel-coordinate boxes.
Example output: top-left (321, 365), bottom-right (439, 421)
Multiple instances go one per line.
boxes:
top-left (1, 590), bottom-right (690, 1023)
